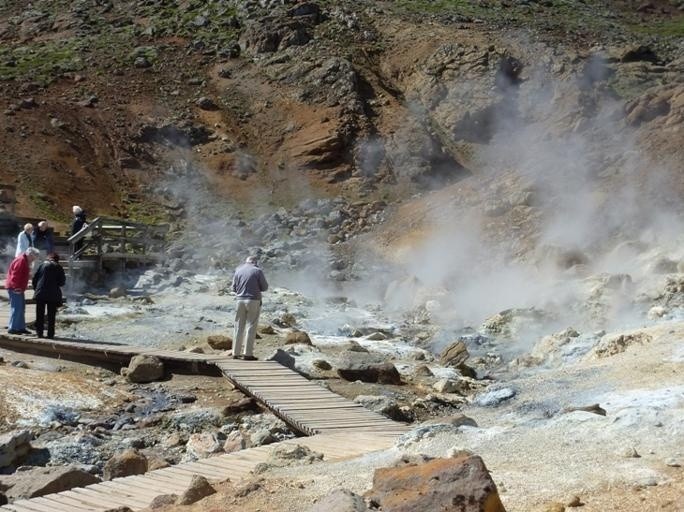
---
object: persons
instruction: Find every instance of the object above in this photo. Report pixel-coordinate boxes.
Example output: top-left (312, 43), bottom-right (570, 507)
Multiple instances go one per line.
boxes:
top-left (68, 205), bottom-right (84, 260)
top-left (13, 222), bottom-right (34, 257)
top-left (30, 252), bottom-right (65, 339)
top-left (30, 220), bottom-right (54, 276)
top-left (3, 246), bottom-right (40, 336)
top-left (228, 255), bottom-right (268, 360)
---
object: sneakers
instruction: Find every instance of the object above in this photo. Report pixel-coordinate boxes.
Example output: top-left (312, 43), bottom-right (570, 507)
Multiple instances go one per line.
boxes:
top-left (8, 328), bottom-right (53, 339)
top-left (244, 356), bottom-right (257, 360)
top-left (233, 356), bottom-right (238, 358)
